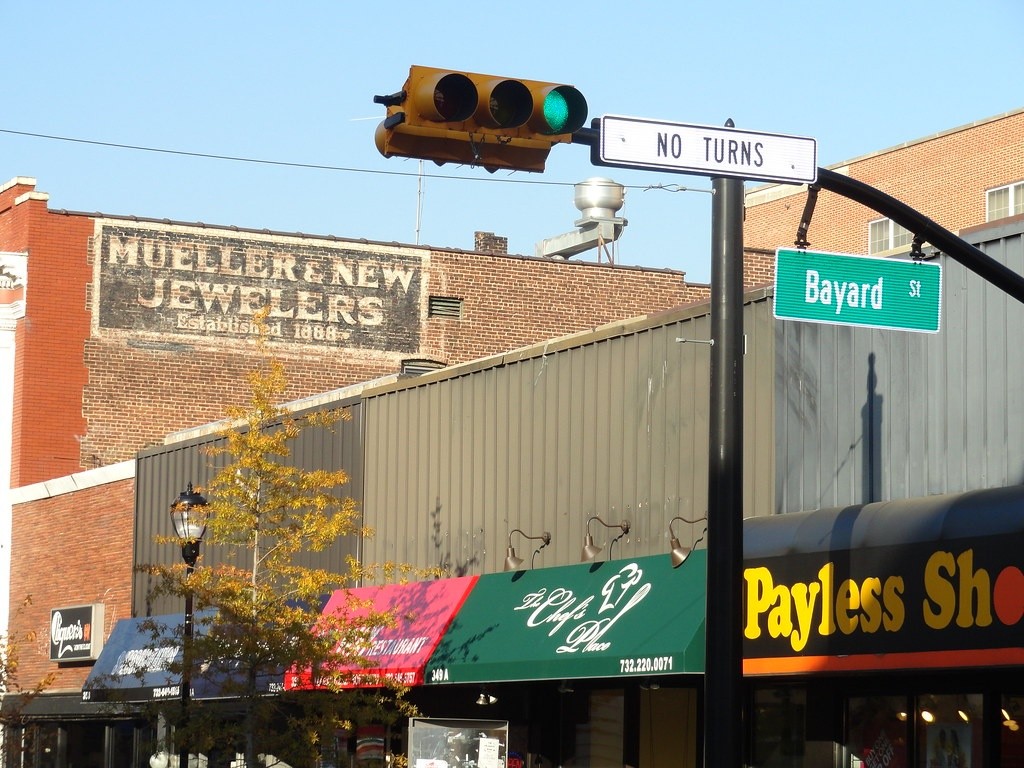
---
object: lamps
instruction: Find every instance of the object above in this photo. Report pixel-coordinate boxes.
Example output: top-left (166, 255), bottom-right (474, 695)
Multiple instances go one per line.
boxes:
top-left (504, 529), bottom-right (551, 572)
top-left (668, 516), bottom-right (708, 568)
top-left (579, 517), bottom-right (631, 564)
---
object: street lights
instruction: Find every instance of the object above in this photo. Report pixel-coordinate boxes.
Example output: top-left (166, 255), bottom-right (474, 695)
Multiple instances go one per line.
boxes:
top-left (168, 480), bottom-right (212, 766)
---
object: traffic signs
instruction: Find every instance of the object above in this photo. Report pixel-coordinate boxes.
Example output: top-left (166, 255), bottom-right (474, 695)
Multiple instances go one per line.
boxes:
top-left (599, 115), bottom-right (818, 184)
top-left (772, 249), bottom-right (944, 334)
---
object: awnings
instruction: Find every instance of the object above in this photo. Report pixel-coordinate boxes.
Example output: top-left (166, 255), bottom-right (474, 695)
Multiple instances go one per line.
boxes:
top-left (424, 546), bottom-right (706, 687)
top-left (284, 574), bottom-right (480, 689)
top-left (184, 593), bottom-right (333, 700)
top-left (81, 605), bottom-right (224, 703)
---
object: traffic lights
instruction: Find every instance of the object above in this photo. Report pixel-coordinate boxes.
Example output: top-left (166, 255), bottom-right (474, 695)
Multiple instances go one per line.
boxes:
top-left (406, 62), bottom-right (592, 148)
